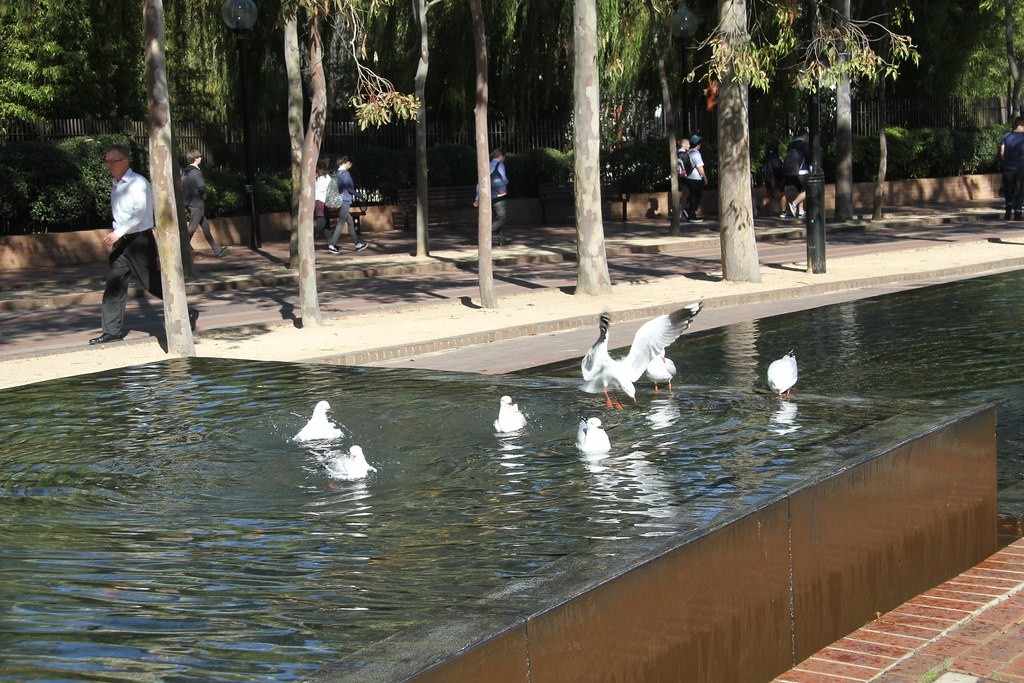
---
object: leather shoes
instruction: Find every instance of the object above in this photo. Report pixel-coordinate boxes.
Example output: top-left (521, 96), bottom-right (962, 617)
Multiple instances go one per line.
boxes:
top-left (189, 311), bottom-right (199, 331)
top-left (89, 333), bottom-right (123, 345)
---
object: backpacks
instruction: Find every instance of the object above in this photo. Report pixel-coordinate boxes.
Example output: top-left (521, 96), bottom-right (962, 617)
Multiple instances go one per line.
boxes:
top-left (783, 140), bottom-right (807, 177)
top-left (325, 170), bottom-right (347, 210)
top-left (677, 150), bottom-right (697, 180)
top-left (489, 161), bottom-right (507, 201)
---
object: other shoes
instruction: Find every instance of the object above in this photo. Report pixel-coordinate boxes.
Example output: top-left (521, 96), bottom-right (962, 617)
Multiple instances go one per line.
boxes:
top-left (798, 211), bottom-right (807, 219)
top-left (355, 243), bottom-right (369, 252)
top-left (216, 245), bottom-right (227, 258)
top-left (754, 200), bottom-right (762, 218)
top-left (682, 209), bottom-right (690, 219)
top-left (499, 240), bottom-right (513, 247)
top-left (326, 244), bottom-right (340, 254)
top-left (690, 216), bottom-right (705, 224)
top-left (787, 202), bottom-right (797, 218)
top-left (1014, 211), bottom-right (1024, 221)
top-left (780, 211), bottom-right (794, 218)
top-left (1004, 205), bottom-right (1012, 219)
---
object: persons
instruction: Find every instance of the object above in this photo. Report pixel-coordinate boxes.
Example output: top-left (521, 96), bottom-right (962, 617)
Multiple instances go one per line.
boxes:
top-left (180, 149), bottom-right (230, 257)
top-left (1000, 115), bottom-right (1024, 220)
top-left (677, 133), bottom-right (709, 222)
top-left (787, 126), bottom-right (810, 218)
top-left (759, 138), bottom-right (793, 218)
top-left (89, 144), bottom-right (199, 344)
top-left (314, 163), bottom-right (340, 248)
top-left (327, 153), bottom-right (369, 254)
top-left (472, 148), bottom-right (512, 245)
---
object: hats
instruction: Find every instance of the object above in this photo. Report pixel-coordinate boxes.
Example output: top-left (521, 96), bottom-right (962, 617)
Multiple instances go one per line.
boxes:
top-left (690, 135), bottom-right (702, 147)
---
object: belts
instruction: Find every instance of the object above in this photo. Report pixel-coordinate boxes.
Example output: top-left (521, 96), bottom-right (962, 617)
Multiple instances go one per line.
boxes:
top-left (122, 228), bottom-right (152, 239)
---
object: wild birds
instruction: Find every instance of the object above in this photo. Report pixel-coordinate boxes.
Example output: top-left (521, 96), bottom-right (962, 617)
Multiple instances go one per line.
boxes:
top-left (578, 452), bottom-right (611, 473)
top-left (293, 399), bottom-right (344, 441)
top-left (578, 301), bottom-right (704, 410)
top-left (492, 394), bottom-right (528, 433)
top-left (325, 444), bottom-right (372, 480)
top-left (767, 347), bottom-right (798, 400)
top-left (644, 348), bottom-right (678, 394)
top-left (575, 419), bottom-right (612, 453)
top-left (640, 397), bottom-right (682, 430)
top-left (763, 402), bottom-right (802, 437)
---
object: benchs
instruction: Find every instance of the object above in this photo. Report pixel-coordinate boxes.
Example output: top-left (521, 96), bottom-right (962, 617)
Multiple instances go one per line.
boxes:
top-left (326, 211), bottom-right (365, 236)
top-left (396, 184), bottom-right (479, 231)
top-left (534, 177), bottom-right (630, 226)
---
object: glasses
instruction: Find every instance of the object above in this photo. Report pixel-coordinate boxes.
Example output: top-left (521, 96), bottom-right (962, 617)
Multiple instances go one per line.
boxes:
top-left (101, 156), bottom-right (128, 165)
